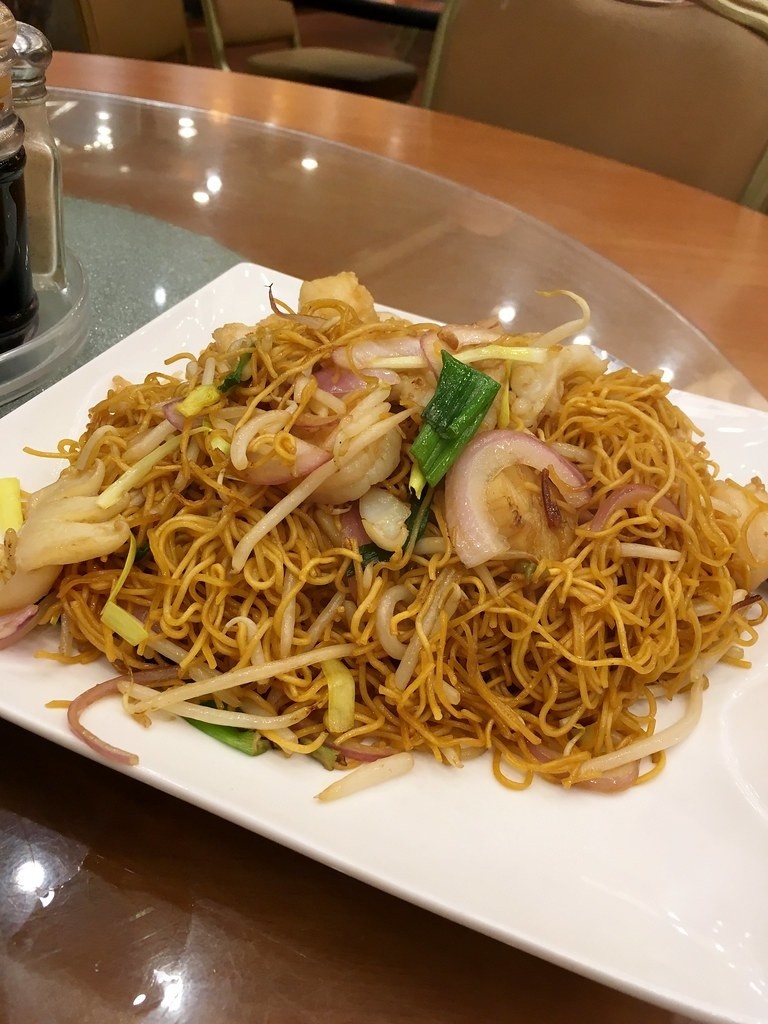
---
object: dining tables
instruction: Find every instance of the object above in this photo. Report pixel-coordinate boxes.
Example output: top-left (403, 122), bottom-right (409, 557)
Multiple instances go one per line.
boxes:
top-left (0, 48), bottom-right (768, 1024)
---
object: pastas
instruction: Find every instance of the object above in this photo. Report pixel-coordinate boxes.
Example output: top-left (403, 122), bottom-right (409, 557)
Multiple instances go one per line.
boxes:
top-left (0, 269), bottom-right (767, 800)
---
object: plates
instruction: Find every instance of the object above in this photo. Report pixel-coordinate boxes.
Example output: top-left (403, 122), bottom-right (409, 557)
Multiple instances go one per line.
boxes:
top-left (0, 258), bottom-right (768, 1024)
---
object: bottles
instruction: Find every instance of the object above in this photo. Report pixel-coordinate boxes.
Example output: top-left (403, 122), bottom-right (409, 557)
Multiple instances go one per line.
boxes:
top-left (0, 5), bottom-right (69, 350)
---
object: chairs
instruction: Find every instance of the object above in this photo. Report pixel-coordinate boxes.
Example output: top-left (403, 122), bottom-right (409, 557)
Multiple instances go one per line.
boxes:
top-left (200, 0), bottom-right (419, 102)
top-left (422, 0), bottom-right (768, 212)
top-left (77, 0), bottom-right (193, 65)
